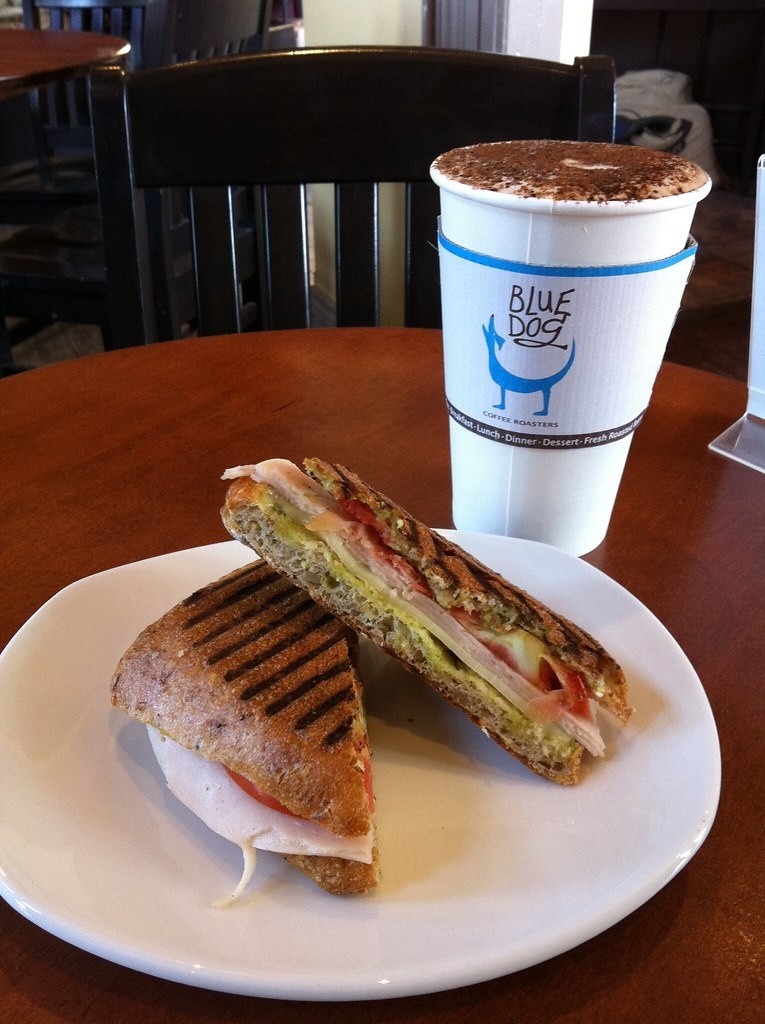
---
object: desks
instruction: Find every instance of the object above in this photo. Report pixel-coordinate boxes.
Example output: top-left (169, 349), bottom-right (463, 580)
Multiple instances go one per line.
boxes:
top-left (0, 27), bottom-right (132, 102)
top-left (0, 324), bottom-right (765, 1024)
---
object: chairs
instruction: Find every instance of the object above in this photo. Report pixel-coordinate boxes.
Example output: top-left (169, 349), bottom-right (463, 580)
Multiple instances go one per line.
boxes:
top-left (0, 0), bottom-right (618, 379)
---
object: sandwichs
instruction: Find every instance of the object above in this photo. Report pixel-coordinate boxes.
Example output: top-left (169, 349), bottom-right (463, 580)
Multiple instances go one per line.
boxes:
top-left (219, 458), bottom-right (632, 791)
top-left (110, 558), bottom-right (381, 904)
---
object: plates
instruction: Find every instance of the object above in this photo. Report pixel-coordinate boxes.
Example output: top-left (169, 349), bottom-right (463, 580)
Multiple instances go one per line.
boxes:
top-left (1, 528), bottom-right (722, 1002)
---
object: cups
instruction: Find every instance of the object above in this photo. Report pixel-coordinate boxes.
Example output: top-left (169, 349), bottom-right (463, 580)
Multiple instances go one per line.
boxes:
top-left (430, 139), bottom-right (713, 563)
